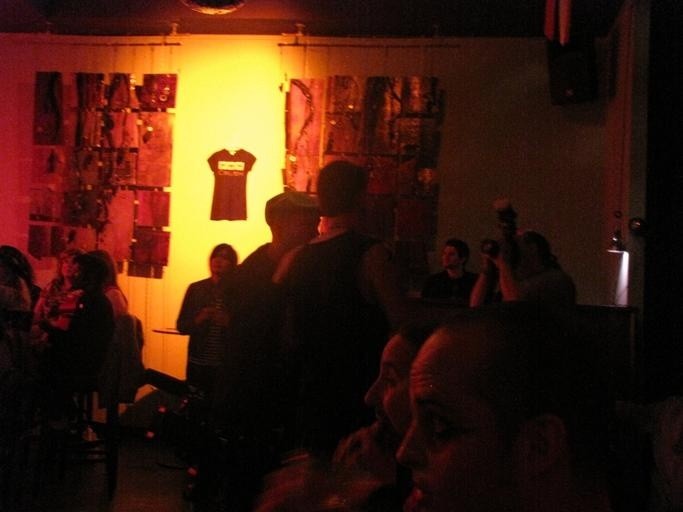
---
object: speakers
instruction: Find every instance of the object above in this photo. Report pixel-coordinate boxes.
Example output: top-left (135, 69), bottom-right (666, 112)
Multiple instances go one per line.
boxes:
top-left (545, 30), bottom-right (600, 106)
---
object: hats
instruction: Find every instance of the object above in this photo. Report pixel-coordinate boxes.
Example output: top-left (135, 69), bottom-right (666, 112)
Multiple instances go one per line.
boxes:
top-left (316, 162), bottom-right (366, 217)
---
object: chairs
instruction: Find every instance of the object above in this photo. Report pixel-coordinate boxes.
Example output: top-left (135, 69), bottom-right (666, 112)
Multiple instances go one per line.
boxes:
top-left (32, 314), bottom-right (144, 505)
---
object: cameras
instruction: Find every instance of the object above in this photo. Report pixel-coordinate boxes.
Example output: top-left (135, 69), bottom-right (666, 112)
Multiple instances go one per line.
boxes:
top-left (480, 239), bottom-right (499, 258)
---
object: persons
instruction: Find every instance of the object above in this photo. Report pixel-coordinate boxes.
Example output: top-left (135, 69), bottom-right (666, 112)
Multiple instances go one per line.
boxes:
top-left (35, 250), bottom-right (113, 440)
top-left (174, 161), bottom-right (418, 509)
top-left (420, 230), bottom-right (578, 308)
top-left (88, 251), bottom-right (128, 319)
top-left (29, 247), bottom-right (84, 348)
top-left (330, 298), bottom-right (653, 511)
top-left (644, 396), bottom-right (682, 512)
